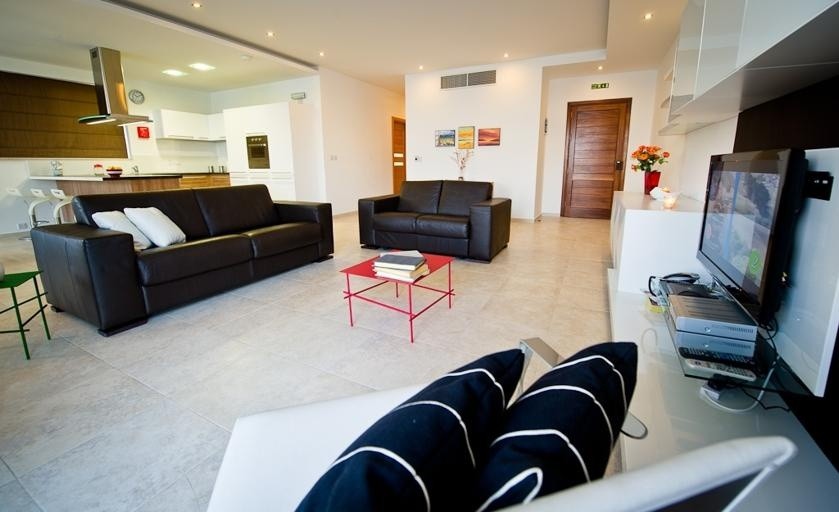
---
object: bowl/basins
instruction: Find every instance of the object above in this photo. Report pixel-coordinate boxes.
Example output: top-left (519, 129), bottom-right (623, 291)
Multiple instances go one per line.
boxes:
top-left (106, 169), bottom-right (122, 178)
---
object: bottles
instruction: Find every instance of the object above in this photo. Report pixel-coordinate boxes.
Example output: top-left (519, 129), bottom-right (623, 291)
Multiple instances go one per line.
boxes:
top-left (94, 164), bottom-right (103, 175)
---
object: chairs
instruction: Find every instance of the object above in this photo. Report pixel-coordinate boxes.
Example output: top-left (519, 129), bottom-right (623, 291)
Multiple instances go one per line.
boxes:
top-left (208, 379), bottom-right (796, 512)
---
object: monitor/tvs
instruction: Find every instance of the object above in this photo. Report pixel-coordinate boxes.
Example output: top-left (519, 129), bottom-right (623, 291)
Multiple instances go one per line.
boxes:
top-left (695, 150), bottom-right (808, 326)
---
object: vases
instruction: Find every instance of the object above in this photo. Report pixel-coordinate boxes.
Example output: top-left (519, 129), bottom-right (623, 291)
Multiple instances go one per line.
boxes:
top-left (644, 171), bottom-right (661, 196)
top-left (458, 177), bottom-right (463, 180)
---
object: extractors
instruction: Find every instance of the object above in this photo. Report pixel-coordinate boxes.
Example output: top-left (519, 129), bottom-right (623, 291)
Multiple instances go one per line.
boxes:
top-left (78, 48), bottom-right (154, 125)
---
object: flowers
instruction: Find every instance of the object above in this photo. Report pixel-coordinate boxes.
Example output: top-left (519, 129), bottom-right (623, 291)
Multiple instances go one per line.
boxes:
top-left (451, 148), bottom-right (477, 177)
top-left (630, 144), bottom-right (671, 172)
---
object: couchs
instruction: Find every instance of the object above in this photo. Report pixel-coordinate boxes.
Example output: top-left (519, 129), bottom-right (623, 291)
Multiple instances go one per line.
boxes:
top-left (28, 184), bottom-right (333, 337)
top-left (358, 180), bottom-right (511, 263)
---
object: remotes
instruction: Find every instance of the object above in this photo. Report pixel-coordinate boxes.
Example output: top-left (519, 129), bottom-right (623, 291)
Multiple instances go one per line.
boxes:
top-left (678, 344), bottom-right (762, 368)
top-left (684, 357), bottom-right (756, 381)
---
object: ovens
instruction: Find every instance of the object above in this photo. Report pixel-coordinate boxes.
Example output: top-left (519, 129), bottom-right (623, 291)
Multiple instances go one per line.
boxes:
top-left (246, 135), bottom-right (269, 168)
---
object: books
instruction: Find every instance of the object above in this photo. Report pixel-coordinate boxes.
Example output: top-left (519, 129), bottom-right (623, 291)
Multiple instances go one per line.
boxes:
top-left (372, 254), bottom-right (430, 282)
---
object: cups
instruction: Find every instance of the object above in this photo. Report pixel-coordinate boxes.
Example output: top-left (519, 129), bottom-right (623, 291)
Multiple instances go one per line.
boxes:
top-left (208, 165), bottom-right (224, 174)
top-left (663, 193), bottom-right (680, 208)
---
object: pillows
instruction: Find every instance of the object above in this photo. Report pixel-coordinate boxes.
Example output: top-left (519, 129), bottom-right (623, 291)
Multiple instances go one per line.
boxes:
top-left (90, 210), bottom-right (153, 251)
top-left (298, 348), bottom-right (527, 511)
top-left (124, 206), bottom-right (188, 248)
top-left (464, 340), bottom-right (639, 512)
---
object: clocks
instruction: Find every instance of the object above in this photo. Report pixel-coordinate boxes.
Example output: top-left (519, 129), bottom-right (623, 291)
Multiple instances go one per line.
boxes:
top-left (128, 89), bottom-right (146, 104)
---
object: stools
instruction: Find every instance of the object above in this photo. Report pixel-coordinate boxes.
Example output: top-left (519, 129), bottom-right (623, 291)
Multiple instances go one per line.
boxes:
top-left (0, 269), bottom-right (50, 360)
top-left (7, 186), bottom-right (74, 240)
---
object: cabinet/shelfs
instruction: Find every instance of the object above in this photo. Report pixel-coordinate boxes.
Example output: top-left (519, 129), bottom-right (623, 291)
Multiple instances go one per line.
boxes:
top-left (654, 0), bottom-right (839, 137)
top-left (154, 108), bottom-right (226, 142)
top-left (609, 189), bottom-right (714, 293)
